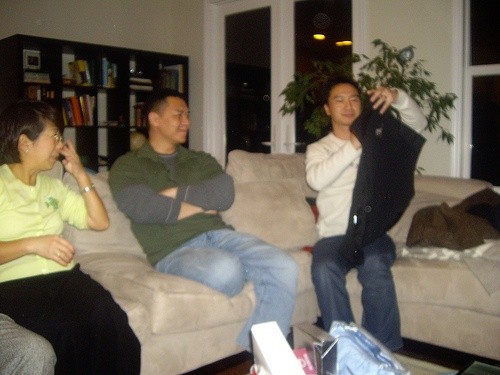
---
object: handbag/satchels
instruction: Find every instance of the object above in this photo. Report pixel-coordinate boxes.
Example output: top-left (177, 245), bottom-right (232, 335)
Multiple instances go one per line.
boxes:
top-left (348, 94), bottom-right (425, 254)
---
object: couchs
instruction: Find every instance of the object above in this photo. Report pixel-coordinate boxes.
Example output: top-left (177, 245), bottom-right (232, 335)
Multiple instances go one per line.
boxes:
top-left (0, 151), bottom-right (499, 375)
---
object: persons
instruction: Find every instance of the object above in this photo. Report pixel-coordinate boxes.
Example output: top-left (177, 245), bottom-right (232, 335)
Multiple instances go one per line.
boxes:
top-left (305, 77), bottom-right (429, 351)
top-left (0, 102), bottom-right (140, 375)
top-left (108, 88), bottom-right (300, 354)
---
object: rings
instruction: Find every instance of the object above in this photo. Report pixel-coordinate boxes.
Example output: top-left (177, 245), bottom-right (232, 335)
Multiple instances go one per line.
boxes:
top-left (379, 94), bottom-right (387, 100)
top-left (58, 250), bottom-right (61, 257)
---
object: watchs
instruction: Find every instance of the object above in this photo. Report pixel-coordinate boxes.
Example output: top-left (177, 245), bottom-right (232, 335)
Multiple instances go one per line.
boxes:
top-left (79, 184), bottom-right (96, 193)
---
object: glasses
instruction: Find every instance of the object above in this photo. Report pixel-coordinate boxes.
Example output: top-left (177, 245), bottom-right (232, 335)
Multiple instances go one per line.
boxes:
top-left (36, 132), bottom-right (65, 144)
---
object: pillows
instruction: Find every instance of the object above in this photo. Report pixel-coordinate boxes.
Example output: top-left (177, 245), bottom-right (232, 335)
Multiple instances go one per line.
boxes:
top-left (61, 170), bottom-right (146, 257)
top-left (219, 178), bottom-right (319, 249)
top-left (388, 190), bottom-right (461, 243)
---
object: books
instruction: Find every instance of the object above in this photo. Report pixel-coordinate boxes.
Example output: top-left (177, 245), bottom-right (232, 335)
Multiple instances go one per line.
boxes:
top-left (21, 49), bottom-right (183, 171)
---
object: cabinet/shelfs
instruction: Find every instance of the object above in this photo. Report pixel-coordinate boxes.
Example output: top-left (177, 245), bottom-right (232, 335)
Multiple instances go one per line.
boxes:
top-left (0, 34), bottom-right (190, 172)
